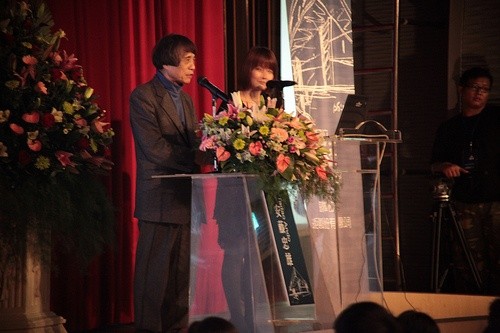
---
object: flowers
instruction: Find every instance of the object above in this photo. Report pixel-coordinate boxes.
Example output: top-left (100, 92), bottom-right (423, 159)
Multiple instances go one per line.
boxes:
top-left (195, 91), bottom-right (344, 212)
top-left (0, 1), bottom-right (122, 175)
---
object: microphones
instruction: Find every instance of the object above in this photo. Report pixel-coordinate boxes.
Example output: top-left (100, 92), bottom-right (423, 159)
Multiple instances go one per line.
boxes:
top-left (266, 80), bottom-right (297, 89)
top-left (198, 76), bottom-right (230, 102)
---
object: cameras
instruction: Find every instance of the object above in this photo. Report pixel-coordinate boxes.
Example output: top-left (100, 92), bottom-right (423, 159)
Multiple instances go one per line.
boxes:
top-left (431, 182), bottom-right (451, 197)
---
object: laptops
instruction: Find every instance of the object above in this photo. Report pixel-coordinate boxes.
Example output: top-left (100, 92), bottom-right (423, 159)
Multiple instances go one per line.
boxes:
top-left (335, 94), bottom-right (370, 137)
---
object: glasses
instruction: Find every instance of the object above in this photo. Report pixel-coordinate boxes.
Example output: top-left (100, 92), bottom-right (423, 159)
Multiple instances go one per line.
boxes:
top-left (463, 83), bottom-right (491, 93)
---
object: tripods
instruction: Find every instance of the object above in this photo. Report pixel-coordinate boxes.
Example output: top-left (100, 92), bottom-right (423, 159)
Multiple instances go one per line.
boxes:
top-left (430, 198), bottom-right (484, 294)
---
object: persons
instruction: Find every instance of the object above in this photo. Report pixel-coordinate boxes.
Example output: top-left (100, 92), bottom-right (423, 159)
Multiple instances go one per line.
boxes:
top-left (187, 299), bottom-right (500, 333)
top-left (213, 46), bottom-right (284, 333)
top-left (426, 68), bottom-right (500, 296)
top-left (130, 34), bottom-right (209, 333)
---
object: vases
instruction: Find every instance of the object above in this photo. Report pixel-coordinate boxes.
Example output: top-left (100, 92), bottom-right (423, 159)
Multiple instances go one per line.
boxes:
top-left (0, 203), bottom-right (69, 333)
